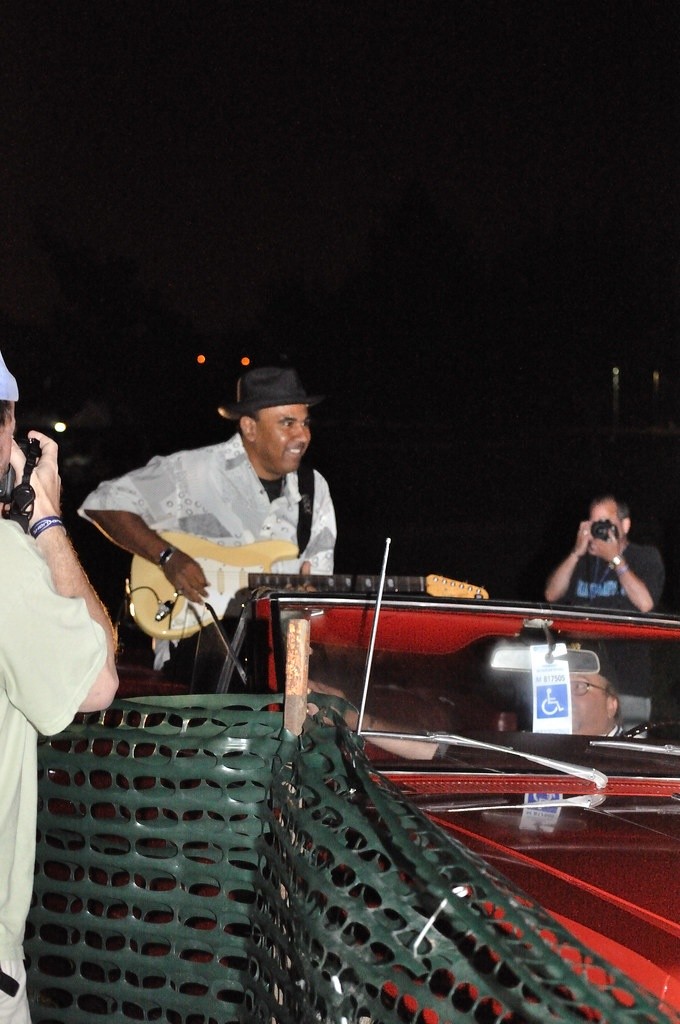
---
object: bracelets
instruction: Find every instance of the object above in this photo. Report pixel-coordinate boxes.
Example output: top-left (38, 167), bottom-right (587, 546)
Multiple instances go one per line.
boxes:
top-left (571, 551), bottom-right (581, 560)
top-left (616, 564), bottom-right (630, 577)
top-left (28, 515), bottom-right (68, 540)
top-left (367, 715), bottom-right (375, 732)
top-left (158, 546), bottom-right (177, 568)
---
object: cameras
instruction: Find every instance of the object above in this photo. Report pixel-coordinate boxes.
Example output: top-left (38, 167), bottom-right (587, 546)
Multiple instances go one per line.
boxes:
top-left (0, 437), bottom-right (34, 503)
top-left (591, 520), bottom-right (619, 542)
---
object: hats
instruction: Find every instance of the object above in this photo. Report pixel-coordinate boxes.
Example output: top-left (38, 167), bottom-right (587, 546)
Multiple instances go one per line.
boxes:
top-left (0, 353), bottom-right (21, 402)
top-left (218, 367), bottom-right (324, 420)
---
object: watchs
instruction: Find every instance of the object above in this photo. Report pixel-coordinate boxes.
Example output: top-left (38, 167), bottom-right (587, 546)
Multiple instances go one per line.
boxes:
top-left (608, 555), bottom-right (626, 570)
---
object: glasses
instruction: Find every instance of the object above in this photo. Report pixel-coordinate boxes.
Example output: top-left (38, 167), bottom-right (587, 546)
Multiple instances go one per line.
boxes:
top-left (571, 681), bottom-right (618, 700)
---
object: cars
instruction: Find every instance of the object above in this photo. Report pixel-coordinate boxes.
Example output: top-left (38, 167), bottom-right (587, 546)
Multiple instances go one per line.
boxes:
top-left (35, 590), bottom-right (680, 1023)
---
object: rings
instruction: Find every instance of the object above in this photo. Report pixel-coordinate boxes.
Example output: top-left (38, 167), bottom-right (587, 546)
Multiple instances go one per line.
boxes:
top-left (306, 709), bottom-right (310, 713)
top-left (177, 590), bottom-right (183, 596)
top-left (307, 688), bottom-right (312, 694)
top-left (583, 530), bottom-right (587, 535)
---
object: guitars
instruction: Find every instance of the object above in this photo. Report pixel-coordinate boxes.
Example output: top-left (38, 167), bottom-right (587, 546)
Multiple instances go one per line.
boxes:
top-left (126, 527), bottom-right (491, 643)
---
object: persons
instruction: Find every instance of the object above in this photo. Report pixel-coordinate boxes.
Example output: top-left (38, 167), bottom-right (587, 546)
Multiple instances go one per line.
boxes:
top-left (77, 368), bottom-right (337, 690)
top-left (306, 640), bottom-right (645, 763)
top-left (0, 351), bottom-right (120, 1024)
top-left (546, 496), bottom-right (665, 740)
